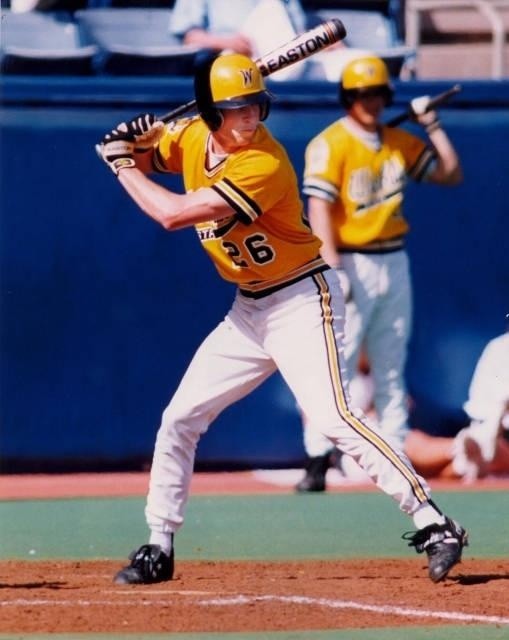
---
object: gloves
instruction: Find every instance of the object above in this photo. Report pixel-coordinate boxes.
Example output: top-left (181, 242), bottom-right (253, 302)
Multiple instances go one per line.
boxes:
top-left (95, 113), bottom-right (165, 175)
top-left (331, 267), bottom-right (352, 303)
top-left (412, 95), bottom-right (441, 135)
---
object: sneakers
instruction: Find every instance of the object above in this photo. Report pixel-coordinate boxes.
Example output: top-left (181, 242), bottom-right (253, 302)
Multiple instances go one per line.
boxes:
top-left (113, 544), bottom-right (173, 583)
top-left (402, 516), bottom-right (469, 584)
top-left (296, 459), bottom-right (331, 490)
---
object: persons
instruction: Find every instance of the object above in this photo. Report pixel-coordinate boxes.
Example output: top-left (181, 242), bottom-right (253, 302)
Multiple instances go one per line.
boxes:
top-left (328, 343), bottom-right (508, 484)
top-left (169, 0), bottom-right (376, 83)
top-left (289, 54), bottom-right (463, 497)
top-left (84, 52), bottom-right (469, 587)
top-left (449, 332), bottom-right (509, 484)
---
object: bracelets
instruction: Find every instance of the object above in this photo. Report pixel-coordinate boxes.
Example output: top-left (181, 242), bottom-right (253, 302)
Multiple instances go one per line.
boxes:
top-left (424, 119), bottom-right (442, 135)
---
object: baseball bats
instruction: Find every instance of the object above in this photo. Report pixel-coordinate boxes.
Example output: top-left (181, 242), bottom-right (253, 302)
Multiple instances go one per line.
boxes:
top-left (156, 20), bottom-right (345, 122)
top-left (388, 86), bottom-right (461, 126)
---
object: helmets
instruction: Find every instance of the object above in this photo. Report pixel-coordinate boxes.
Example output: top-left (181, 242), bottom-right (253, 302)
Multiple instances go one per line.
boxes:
top-left (209, 53), bottom-right (277, 110)
top-left (337, 56), bottom-right (394, 109)
